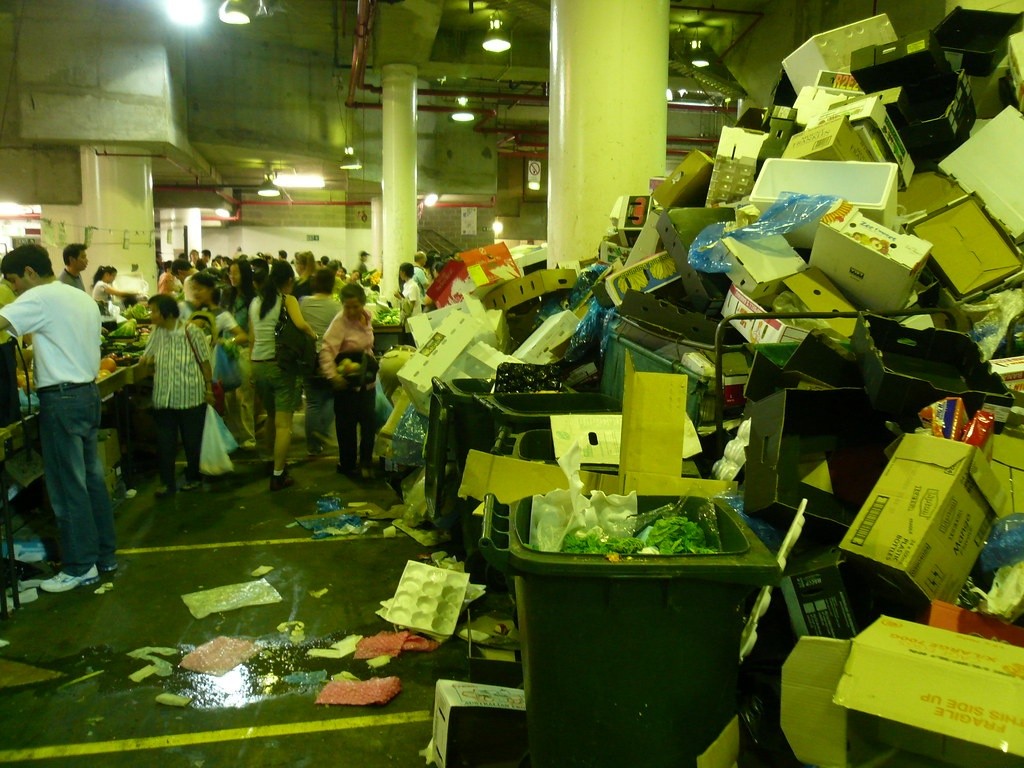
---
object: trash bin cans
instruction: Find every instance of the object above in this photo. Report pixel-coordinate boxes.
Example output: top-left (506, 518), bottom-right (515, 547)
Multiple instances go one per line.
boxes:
top-left (471, 392), bottom-right (624, 425)
top-left (488, 425), bottom-right (702, 492)
top-left (421, 371), bottom-right (574, 551)
top-left (481, 492), bottom-right (785, 768)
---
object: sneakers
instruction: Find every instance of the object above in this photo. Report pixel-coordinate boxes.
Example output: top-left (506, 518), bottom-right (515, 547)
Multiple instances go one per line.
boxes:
top-left (41, 562), bottom-right (101, 591)
top-left (183, 477), bottom-right (206, 490)
top-left (155, 481), bottom-right (177, 497)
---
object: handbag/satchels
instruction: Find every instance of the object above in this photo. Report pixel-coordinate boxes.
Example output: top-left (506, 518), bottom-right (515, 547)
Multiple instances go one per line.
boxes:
top-left (209, 379), bottom-right (226, 416)
top-left (274, 292), bottom-right (316, 376)
top-left (0, 337), bottom-right (33, 430)
top-left (199, 401), bottom-right (238, 476)
top-left (213, 342), bottom-right (242, 391)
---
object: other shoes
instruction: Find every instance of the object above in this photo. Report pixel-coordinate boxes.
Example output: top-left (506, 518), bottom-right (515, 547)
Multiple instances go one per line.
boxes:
top-left (307, 445), bottom-right (324, 456)
top-left (271, 472), bottom-right (294, 491)
top-left (337, 463), bottom-right (356, 477)
top-left (361, 466), bottom-right (375, 480)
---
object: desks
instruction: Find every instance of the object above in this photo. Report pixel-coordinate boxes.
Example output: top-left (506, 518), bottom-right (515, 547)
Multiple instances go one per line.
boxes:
top-left (0, 362), bottom-right (142, 620)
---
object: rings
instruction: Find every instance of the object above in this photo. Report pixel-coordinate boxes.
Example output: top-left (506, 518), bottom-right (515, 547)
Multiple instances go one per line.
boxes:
top-left (210, 400), bottom-right (213, 402)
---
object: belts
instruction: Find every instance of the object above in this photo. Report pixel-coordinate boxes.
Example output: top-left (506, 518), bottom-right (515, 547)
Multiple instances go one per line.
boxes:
top-left (38, 380), bottom-right (87, 392)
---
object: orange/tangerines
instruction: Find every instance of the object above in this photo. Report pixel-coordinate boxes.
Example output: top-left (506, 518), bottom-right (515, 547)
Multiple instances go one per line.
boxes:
top-left (16, 367), bottom-right (34, 391)
top-left (100, 358), bottom-right (116, 372)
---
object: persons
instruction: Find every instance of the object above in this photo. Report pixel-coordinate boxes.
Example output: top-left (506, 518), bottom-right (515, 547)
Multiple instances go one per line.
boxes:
top-left (320, 283), bottom-right (379, 482)
top-left (156, 249), bottom-right (380, 457)
top-left (138, 294), bottom-right (215, 497)
top-left (413, 250), bottom-right (465, 315)
top-left (0, 275), bottom-right (34, 373)
top-left (57, 244), bottom-right (88, 295)
top-left (249, 260), bottom-right (319, 491)
top-left (92, 264), bottom-right (142, 316)
top-left (393, 262), bottom-right (423, 348)
top-left (0, 244), bottom-right (120, 592)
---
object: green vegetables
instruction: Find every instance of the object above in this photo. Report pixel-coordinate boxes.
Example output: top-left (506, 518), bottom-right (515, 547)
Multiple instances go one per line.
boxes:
top-left (558, 516), bottom-right (720, 555)
top-left (376, 310), bottom-right (401, 326)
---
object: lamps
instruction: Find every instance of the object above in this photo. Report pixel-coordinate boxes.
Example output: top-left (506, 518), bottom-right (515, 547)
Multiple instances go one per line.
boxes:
top-left (482, 10), bottom-right (512, 51)
top-left (339, 147), bottom-right (361, 169)
top-left (452, 96), bottom-right (474, 121)
top-left (690, 41), bottom-right (708, 67)
top-left (257, 171), bottom-right (280, 196)
top-left (219, 0), bottom-right (250, 24)
top-left (215, 202), bottom-right (233, 216)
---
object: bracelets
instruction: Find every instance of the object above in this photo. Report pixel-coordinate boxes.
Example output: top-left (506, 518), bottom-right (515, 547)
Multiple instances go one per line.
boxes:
top-left (206, 379), bottom-right (213, 384)
top-left (315, 334), bottom-right (319, 340)
top-left (206, 391), bottom-right (214, 395)
top-left (231, 335), bottom-right (236, 342)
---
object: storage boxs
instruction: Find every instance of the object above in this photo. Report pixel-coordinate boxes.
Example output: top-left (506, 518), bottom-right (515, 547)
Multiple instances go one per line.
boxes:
top-left (96, 428), bottom-right (119, 471)
top-left (102, 468), bottom-right (121, 495)
top-left (369, 0), bottom-right (1024, 768)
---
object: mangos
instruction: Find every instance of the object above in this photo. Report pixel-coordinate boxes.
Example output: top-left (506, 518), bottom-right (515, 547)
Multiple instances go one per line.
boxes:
top-left (337, 357), bottom-right (360, 373)
